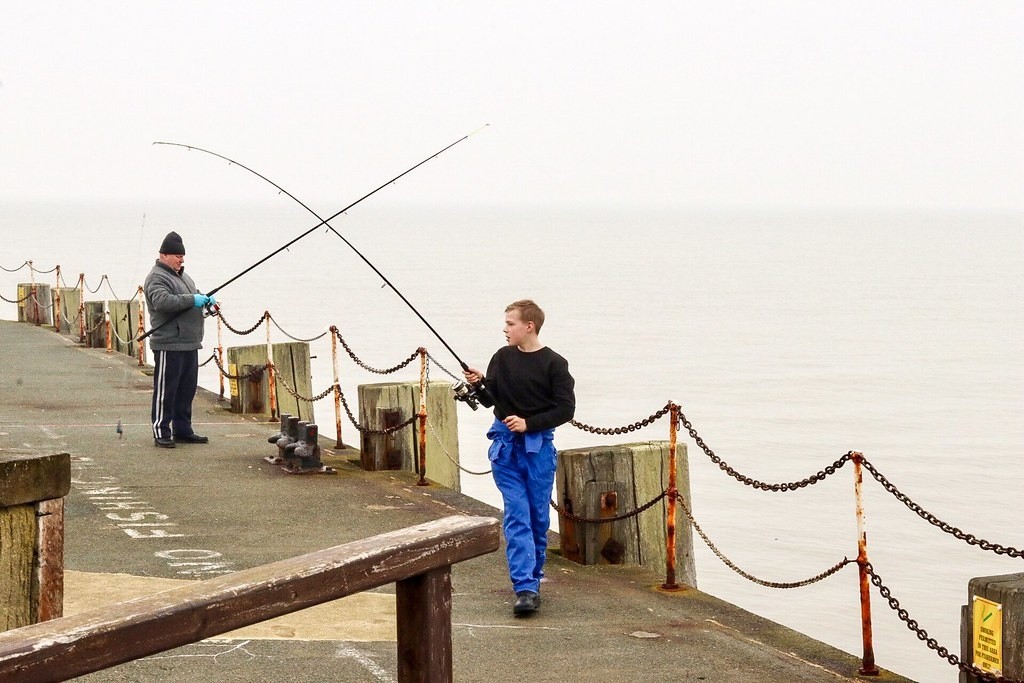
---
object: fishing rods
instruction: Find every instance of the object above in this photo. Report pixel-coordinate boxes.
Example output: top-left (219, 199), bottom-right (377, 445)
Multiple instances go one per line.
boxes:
top-left (151, 139), bottom-right (525, 437)
top-left (136, 123), bottom-right (491, 343)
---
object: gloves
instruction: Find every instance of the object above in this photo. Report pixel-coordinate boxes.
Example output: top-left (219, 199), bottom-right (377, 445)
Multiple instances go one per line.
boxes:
top-left (208, 296), bottom-right (216, 304)
top-left (194, 294), bottom-right (209, 307)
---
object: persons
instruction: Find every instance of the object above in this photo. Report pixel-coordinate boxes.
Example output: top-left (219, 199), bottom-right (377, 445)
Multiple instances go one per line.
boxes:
top-left (144, 232), bottom-right (216, 448)
top-left (462, 300), bottom-right (575, 614)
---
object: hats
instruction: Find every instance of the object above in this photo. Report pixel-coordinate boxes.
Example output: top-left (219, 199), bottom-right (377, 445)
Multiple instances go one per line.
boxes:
top-left (159, 231), bottom-right (185, 255)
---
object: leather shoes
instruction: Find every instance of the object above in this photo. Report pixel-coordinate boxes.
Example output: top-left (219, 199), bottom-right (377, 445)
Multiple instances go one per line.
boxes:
top-left (513, 590), bottom-right (539, 613)
top-left (173, 432), bottom-right (208, 443)
top-left (155, 438), bottom-right (175, 448)
